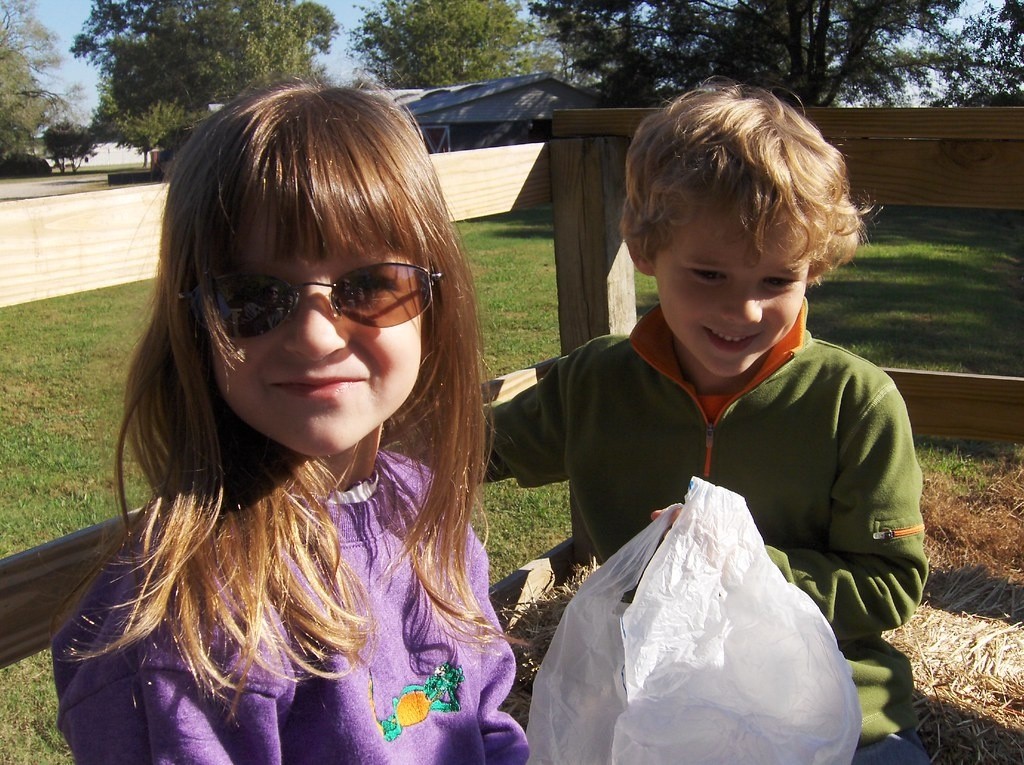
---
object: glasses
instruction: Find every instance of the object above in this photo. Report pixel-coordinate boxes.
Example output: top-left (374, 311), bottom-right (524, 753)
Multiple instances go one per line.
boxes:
top-left (177, 263), bottom-right (442, 338)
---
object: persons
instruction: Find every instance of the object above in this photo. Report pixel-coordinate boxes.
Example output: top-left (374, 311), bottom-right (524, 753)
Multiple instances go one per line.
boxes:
top-left (458, 82), bottom-right (931, 765)
top-left (51, 75), bottom-right (531, 765)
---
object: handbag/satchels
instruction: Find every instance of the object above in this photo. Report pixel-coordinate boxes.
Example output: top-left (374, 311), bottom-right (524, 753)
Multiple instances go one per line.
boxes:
top-left (524, 476), bottom-right (862, 765)
